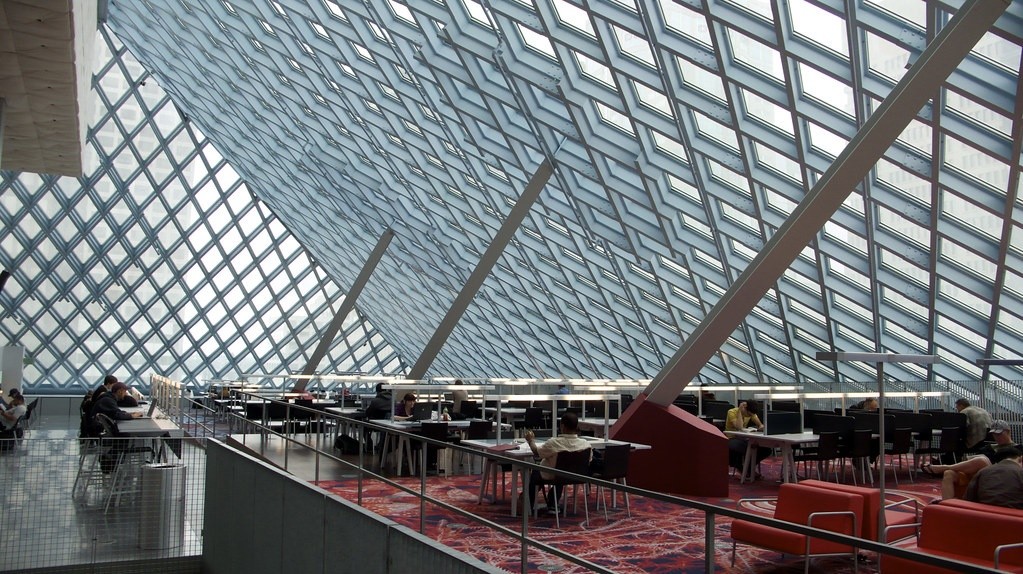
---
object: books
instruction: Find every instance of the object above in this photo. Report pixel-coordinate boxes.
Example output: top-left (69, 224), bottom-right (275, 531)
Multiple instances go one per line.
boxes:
top-left (742, 427), bottom-right (762, 432)
top-left (0, 403), bottom-right (7, 412)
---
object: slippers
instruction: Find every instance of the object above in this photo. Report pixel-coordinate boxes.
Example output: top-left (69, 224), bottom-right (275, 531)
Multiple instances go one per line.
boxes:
top-left (920, 463), bottom-right (936, 475)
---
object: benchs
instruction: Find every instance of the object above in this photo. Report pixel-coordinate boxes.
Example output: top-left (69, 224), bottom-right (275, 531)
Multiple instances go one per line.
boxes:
top-left (730, 479), bottom-right (1023, 574)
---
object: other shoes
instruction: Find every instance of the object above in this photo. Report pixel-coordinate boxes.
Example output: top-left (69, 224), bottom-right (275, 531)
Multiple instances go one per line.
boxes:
top-left (735, 469), bottom-right (752, 479)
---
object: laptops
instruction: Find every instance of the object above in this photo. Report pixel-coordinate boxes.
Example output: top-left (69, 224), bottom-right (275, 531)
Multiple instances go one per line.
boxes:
top-left (133, 397), bottom-right (157, 419)
top-left (405, 402), bottom-right (433, 421)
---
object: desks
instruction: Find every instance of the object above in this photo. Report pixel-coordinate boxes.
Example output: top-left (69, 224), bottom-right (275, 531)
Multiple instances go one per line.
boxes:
top-left (723, 428), bottom-right (942, 484)
top-left (114, 400), bottom-right (180, 507)
top-left (214, 400), bottom-right (652, 517)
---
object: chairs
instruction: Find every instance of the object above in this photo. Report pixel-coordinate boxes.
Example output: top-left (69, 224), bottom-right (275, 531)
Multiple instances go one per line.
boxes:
top-left (247, 399), bottom-right (631, 529)
top-left (0, 397), bottom-right (39, 449)
top-left (673, 395), bottom-right (994, 488)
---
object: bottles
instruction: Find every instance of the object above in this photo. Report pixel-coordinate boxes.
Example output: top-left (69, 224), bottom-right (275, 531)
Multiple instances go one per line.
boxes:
top-left (443, 407), bottom-right (448, 421)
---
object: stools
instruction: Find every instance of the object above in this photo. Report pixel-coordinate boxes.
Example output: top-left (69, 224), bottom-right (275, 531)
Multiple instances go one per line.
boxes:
top-left (71, 406), bottom-right (151, 515)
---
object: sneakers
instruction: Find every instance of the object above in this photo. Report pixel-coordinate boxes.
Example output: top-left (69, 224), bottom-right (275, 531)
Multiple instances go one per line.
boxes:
top-left (547, 504), bottom-right (561, 514)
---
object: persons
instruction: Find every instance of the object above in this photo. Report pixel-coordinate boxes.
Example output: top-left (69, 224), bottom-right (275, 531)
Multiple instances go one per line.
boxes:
top-left (79, 375), bottom-right (143, 473)
top-left (849, 398), bottom-right (878, 411)
top-left (394, 393), bottom-right (422, 422)
top-left (451, 380), bottom-right (468, 412)
top-left (334, 388), bottom-right (350, 401)
top-left (360, 382), bottom-right (391, 454)
top-left (0, 388), bottom-right (26, 452)
top-left (517, 413), bottom-right (593, 516)
top-left (216, 386), bottom-right (229, 400)
top-left (725, 400), bottom-right (771, 481)
top-left (549, 384), bottom-right (571, 394)
top-left (921, 398), bottom-right (1023, 512)
top-left (696, 383), bottom-right (716, 401)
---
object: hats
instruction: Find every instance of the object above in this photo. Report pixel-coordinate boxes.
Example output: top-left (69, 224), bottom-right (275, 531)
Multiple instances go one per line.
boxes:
top-left (988, 419), bottom-right (1011, 434)
top-left (12, 395), bottom-right (24, 401)
top-left (403, 393), bottom-right (417, 402)
top-left (7, 388), bottom-right (18, 396)
top-left (376, 383), bottom-right (386, 392)
top-left (993, 446), bottom-right (1022, 463)
top-left (111, 382), bottom-right (131, 392)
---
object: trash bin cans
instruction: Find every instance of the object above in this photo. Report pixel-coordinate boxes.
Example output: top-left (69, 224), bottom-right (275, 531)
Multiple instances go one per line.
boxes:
top-left (140, 462), bottom-right (187, 550)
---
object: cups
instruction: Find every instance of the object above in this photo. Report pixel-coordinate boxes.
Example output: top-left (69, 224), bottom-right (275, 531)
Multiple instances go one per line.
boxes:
top-left (431, 411), bottom-right (438, 421)
